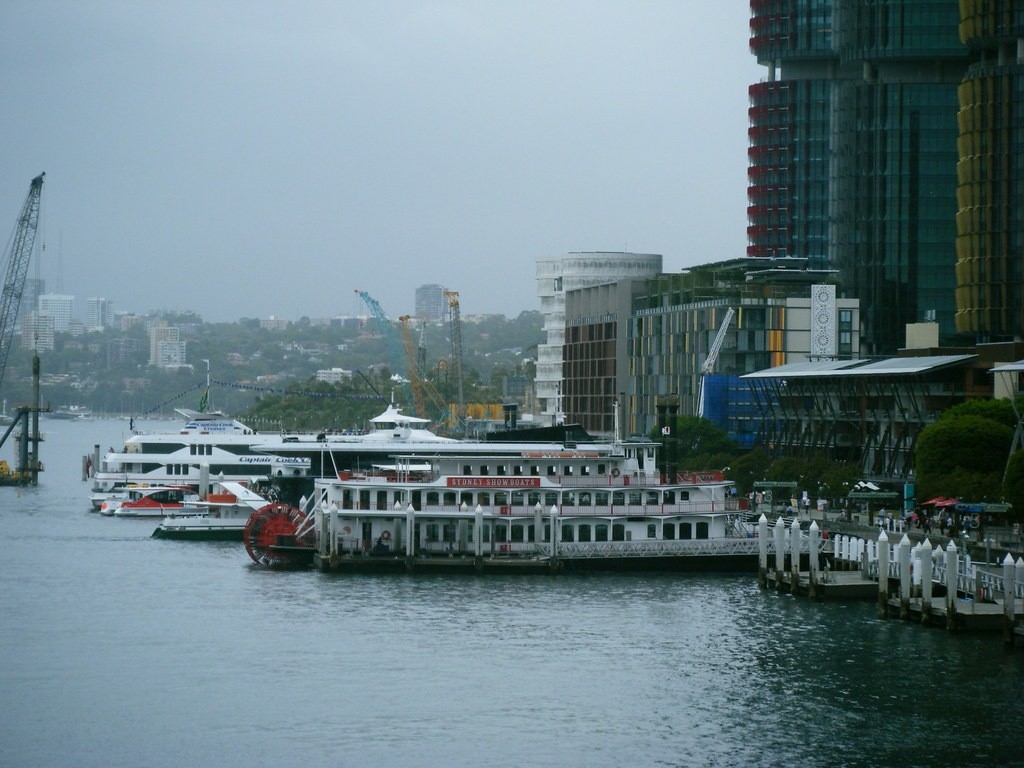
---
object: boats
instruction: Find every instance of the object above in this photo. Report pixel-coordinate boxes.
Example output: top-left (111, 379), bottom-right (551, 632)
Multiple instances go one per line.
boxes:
top-left (88, 403), bottom-right (736, 574)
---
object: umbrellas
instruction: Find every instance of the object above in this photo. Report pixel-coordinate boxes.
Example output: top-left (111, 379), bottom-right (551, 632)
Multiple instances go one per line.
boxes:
top-left (935, 498), bottom-right (959, 525)
top-left (922, 496), bottom-right (950, 525)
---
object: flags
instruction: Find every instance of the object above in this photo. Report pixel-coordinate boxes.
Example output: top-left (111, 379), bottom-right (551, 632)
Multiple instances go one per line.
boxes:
top-left (199, 389), bottom-right (208, 413)
top-left (86, 453), bottom-right (93, 478)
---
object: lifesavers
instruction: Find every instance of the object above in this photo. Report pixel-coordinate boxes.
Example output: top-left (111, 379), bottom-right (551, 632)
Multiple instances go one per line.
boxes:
top-left (611, 468), bottom-right (620, 477)
top-left (381, 530), bottom-right (390, 540)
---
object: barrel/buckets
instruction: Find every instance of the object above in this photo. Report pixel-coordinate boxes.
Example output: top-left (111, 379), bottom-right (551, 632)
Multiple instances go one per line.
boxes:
top-left (339, 471), bottom-right (348, 481)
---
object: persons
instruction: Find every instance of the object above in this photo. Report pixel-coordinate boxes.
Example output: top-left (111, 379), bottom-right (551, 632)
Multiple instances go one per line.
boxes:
top-left (731, 485), bottom-right (737, 499)
top-left (317, 430), bottom-right (326, 442)
top-left (878, 508), bottom-right (886, 520)
top-left (248, 430), bottom-right (251, 434)
top-left (905, 508), bottom-right (980, 541)
top-left (804, 497), bottom-right (810, 514)
top-left (253, 428), bottom-right (257, 434)
top-left (887, 512), bottom-right (894, 521)
top-left (840, 508), bottom-right (845, 520)
